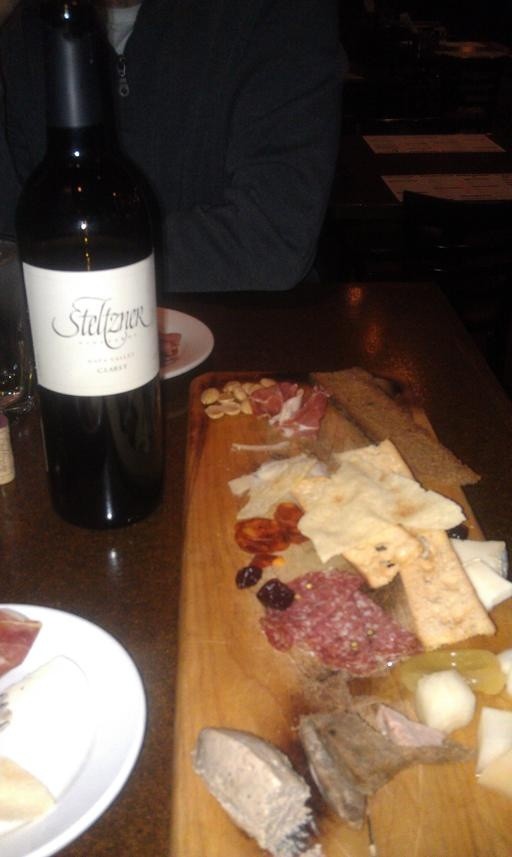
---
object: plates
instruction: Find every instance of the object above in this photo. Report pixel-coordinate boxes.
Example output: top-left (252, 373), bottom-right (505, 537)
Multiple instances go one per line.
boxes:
top-left (1, 605), bottom-right (149, 857)
top-left (161, 306), bottom-right (220, 381)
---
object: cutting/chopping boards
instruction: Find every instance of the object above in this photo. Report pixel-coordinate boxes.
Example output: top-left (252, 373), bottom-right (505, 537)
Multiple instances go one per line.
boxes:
top-left (171, 368), bottom-right (512, 854)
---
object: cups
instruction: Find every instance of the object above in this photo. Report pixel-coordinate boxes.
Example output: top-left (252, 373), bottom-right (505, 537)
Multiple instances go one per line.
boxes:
top-left (1, 331), bottom-right (37, 415)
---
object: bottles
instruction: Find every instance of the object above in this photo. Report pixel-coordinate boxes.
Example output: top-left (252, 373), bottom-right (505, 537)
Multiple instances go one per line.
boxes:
top-left (8, 0), bottom-right (167, 533)
